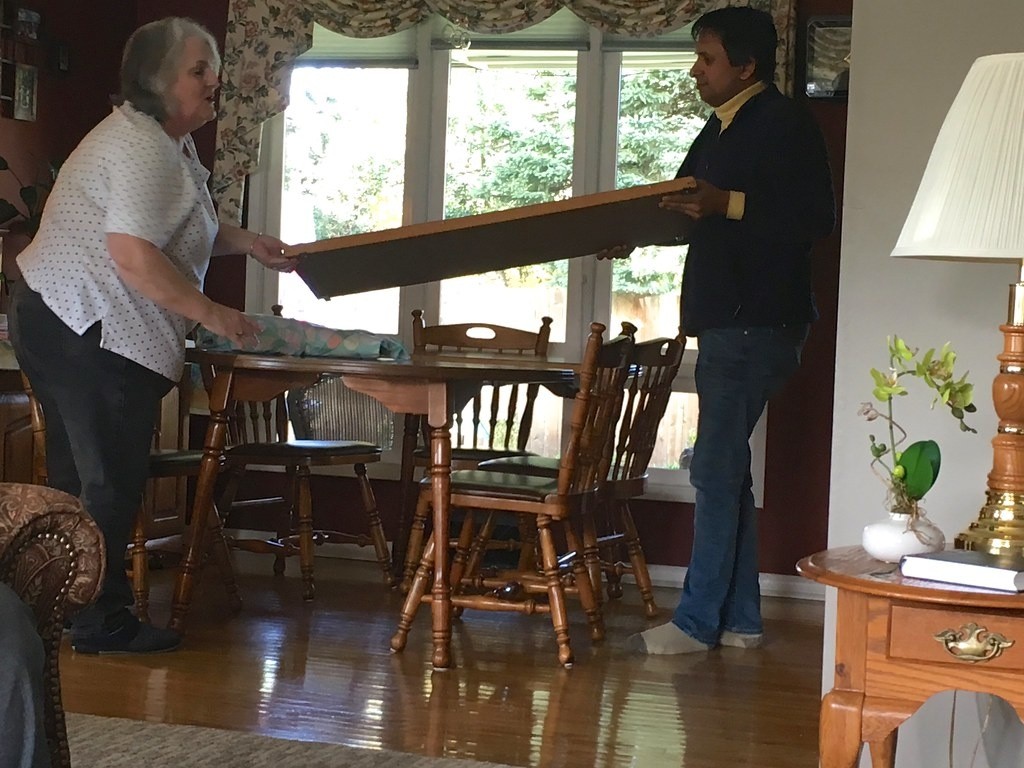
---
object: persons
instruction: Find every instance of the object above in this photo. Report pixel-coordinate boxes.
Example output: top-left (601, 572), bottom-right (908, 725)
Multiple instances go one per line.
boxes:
top-left (8, 18), bottom-right (301, 657)
top-left (595, 6), bottom-right (837, 655)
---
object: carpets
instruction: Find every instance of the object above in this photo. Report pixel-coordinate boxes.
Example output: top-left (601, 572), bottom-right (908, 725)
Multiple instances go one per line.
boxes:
top-left (61, 709), bottom-right (522, 768)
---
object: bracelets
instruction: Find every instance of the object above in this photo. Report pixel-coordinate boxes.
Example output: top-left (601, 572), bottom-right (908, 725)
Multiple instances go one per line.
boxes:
top-left (250, 232), bottom-right (263, 258)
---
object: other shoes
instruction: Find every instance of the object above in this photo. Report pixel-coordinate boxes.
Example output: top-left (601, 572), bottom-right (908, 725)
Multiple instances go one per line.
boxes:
top-left (70, 610), bottom-right (185, 655)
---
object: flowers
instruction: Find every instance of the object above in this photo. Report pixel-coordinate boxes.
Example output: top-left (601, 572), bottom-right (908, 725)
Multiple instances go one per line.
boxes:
top-left (857, 336), bottom-right (977, 515)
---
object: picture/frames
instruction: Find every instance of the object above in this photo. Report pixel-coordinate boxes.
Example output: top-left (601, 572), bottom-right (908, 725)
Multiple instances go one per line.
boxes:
top-left (13, 62), bottom-right (40, 122)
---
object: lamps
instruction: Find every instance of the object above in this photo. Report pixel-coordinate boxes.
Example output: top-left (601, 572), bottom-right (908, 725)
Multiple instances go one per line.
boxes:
top-left (892, 48), bottom-right (1024, 588)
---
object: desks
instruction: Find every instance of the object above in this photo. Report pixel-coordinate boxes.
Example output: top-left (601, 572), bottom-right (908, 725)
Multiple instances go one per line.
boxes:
top-left (176, 345), bottom-right (582, 671)
top-left (796, 546), bottom-right (1024, 767)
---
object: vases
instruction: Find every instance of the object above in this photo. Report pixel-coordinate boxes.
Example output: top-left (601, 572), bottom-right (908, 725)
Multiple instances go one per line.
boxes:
top-left (862, 515), bottom-right (947, 562)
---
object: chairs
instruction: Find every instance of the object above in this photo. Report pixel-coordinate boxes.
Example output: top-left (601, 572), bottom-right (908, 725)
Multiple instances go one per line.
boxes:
top-left (17, 301), bottom-right (695, 670)
